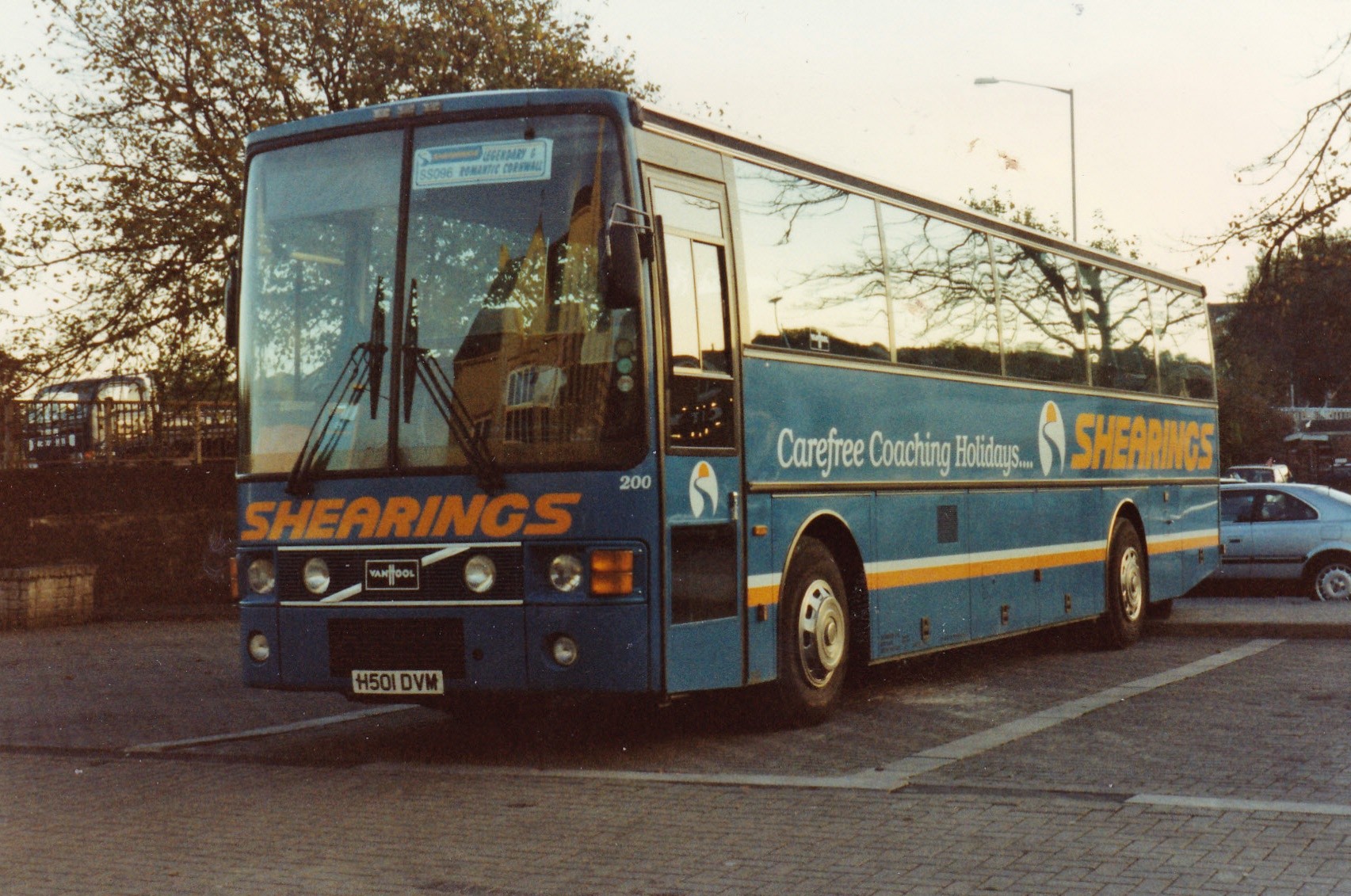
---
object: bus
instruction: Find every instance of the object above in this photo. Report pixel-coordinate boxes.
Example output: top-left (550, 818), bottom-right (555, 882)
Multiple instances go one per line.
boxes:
top-left (222, 85), bottom-right (1223, 729)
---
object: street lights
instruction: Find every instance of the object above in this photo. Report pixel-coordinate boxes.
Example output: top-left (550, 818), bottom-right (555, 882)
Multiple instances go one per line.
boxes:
top-left (973, 74), bottom-right (1079, 242)
top-left (768, 296), bottom-right (784, 348)
top-left (285, 252), bottom-right (347, 399)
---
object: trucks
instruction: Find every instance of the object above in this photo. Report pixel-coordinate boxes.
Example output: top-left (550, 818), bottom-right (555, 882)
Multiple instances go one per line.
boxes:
top-left (16, 370), bottom-right (239, 472)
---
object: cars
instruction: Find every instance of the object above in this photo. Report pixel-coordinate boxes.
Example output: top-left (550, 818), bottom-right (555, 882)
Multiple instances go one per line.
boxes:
top-left (1226, 462), bottom-right (1298, 520)
top-left (1198, 482), bottom-right (1351, 603)
